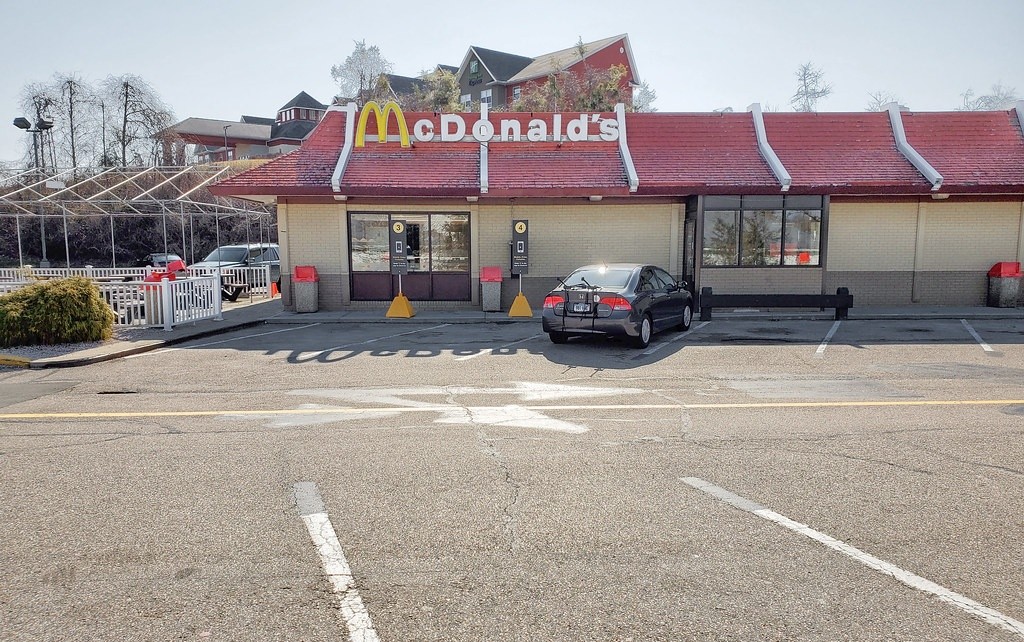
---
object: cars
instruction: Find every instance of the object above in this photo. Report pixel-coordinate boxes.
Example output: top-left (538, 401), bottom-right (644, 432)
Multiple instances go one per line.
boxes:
top-left (135, 253), bottom-right (184, 276)
top-left (542, 262), bottom-right (695, 349)
top-left (187, 243), bottom-right (282, 301)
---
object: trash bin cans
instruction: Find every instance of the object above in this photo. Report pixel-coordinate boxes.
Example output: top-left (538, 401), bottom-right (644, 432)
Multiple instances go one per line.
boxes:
top-left (986, 260), bottom-right (1023, 308)
top-left (479, 264), bottom-right (503, 311)
top-left (143, 272), bottom-right (177, 325)
top-left (292, 265), bottom-right (321, 313)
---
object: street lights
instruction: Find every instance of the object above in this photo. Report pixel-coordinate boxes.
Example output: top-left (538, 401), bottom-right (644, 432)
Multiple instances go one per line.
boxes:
top-left (13, 117), bottom-right (53, 261)
